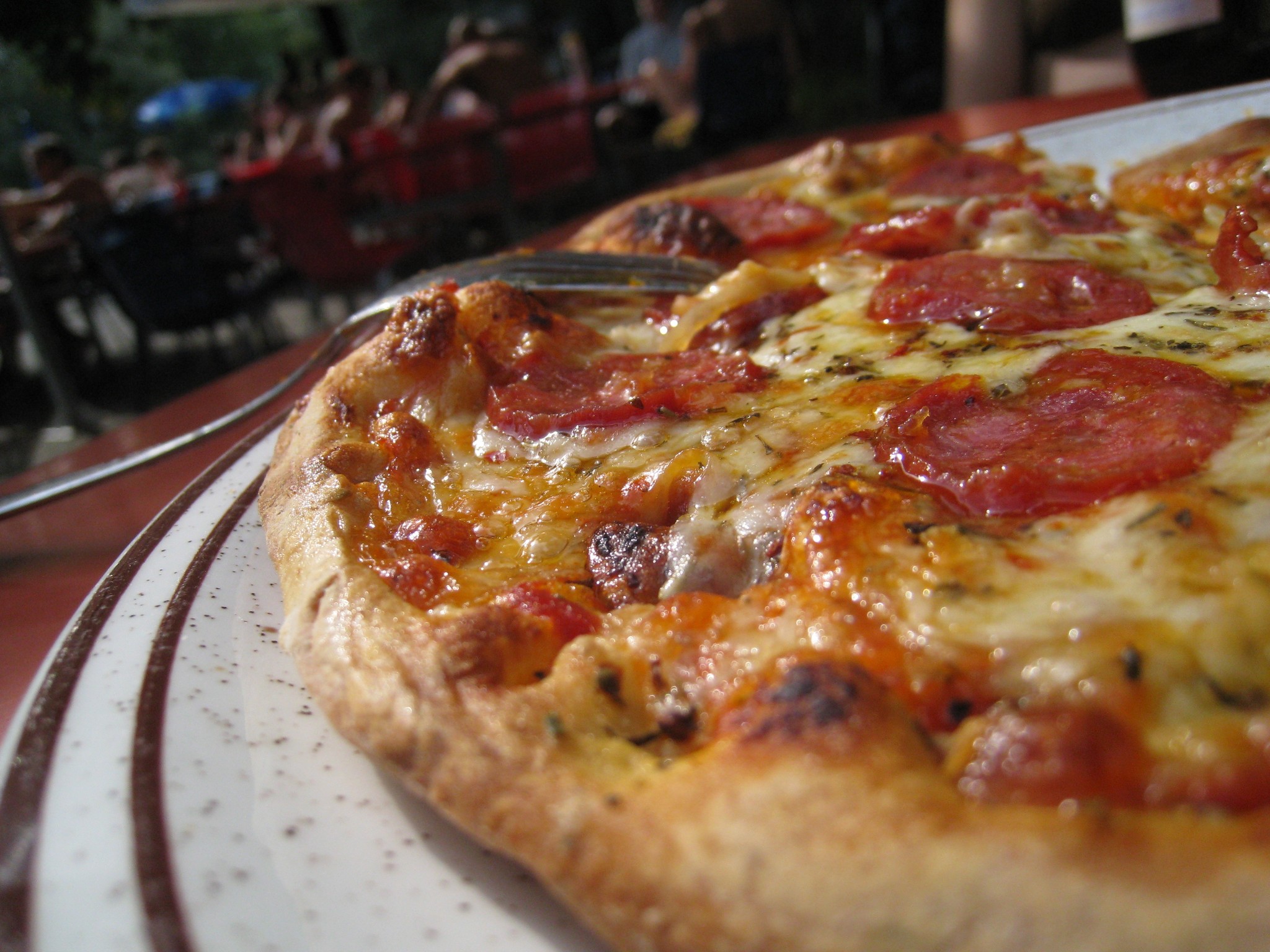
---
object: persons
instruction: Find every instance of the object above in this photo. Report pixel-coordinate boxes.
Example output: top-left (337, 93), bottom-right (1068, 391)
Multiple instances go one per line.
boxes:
top-left (640, 0), bottom-right (797, 167)
top-left (0, 0), bottom-right (682, 255)
top-left (1119, 0), bottom-right (1270, 100)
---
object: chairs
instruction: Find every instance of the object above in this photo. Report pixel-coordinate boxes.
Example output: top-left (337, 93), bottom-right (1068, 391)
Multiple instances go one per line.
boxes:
top-left (93, 80), bottom-right (624, 381)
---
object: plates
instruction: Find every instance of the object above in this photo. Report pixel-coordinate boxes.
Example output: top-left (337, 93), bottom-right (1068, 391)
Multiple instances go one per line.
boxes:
top-left (0, 79), bottom-right (1270, 951)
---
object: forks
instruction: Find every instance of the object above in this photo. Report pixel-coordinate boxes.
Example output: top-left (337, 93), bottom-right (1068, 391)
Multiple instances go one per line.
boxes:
top-left (0, 252), bottom-right (719, 522)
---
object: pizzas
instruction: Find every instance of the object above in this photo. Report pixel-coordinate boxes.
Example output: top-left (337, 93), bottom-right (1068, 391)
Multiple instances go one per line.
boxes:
top-left (262, 127), bottom-right (1270, 952)
